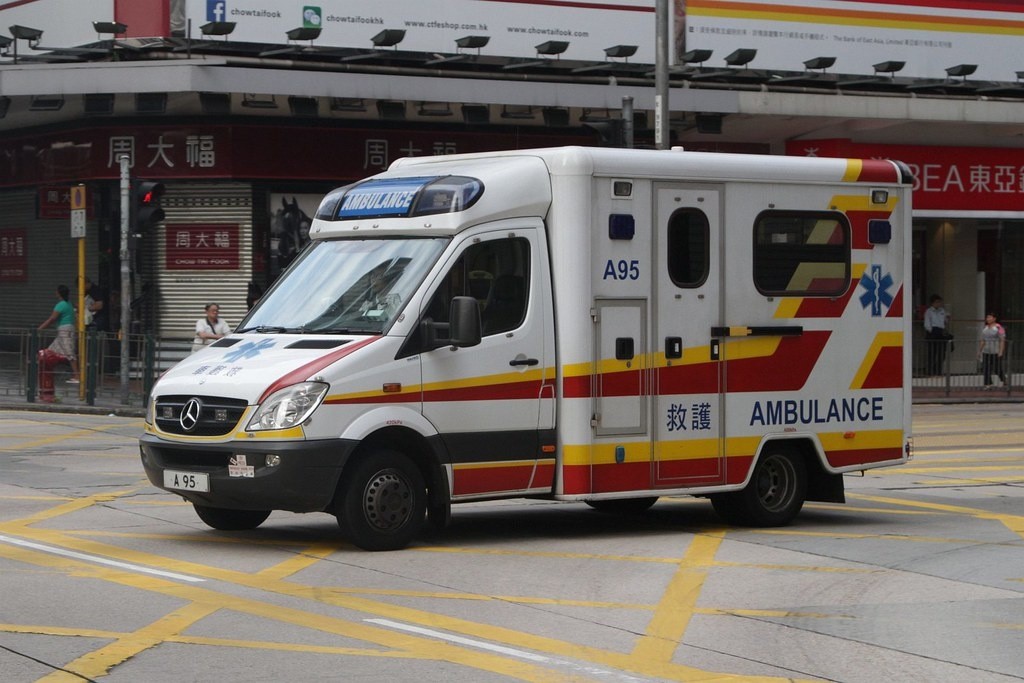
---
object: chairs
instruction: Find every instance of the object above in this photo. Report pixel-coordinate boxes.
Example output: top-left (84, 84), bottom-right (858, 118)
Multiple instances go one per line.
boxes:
top-left (484, 275), bottom-right (525, 332)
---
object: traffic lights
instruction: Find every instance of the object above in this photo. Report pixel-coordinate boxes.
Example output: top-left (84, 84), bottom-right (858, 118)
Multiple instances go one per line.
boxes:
top-left (136, 203), bottom-right (164, 230)
top-left (135, 179), bottom-right (163, 203)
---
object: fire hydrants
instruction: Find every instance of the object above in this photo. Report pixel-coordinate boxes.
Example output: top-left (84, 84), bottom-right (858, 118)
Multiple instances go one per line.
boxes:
top-left (37, 347), bottom-right (63, 404)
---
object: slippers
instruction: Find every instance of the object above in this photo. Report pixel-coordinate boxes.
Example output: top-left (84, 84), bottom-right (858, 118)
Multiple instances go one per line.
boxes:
top-left (65, 378), bottom-right (79, 384)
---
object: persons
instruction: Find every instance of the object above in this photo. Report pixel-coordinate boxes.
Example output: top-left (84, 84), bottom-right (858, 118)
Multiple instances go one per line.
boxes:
top-left (75, 276), bottom-right (108, 366)
top-left (924, 295), bottom-right (955, 376)
top-left (37, 285), bottom-right (80, 383)
top-left (976, 313), bottom-right (1010, 393)
top-left (190, 303), bottom-right (233, 356)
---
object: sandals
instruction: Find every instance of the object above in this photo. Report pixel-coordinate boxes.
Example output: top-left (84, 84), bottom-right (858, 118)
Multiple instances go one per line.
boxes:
top-left (1001, 383), bottom-right (1010, 391)
top-left (983, 383), bottom-right (991, 391)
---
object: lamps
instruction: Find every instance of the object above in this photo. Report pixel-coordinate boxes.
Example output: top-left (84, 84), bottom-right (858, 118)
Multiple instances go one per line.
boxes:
top-left (370, 28), bottom-right (407, 51)
top-left (241, 93), bottom-right (728, 134)
top-left (0, 96), bottom-right (12, 119)
top-left (79, 94), bottom-right (116, 115)
top-left (134, 92), bottom-right (167, 115)
top-left (723, 48), bottom-right (757, 69)
top-left (285, 27), bottom-right (323, 45)
top-left (679, 49), bottom-right (714, 67)
top-left (803, 57), bottom-right (837, 74)
top-left (28, 95), bottom-right (64, 111)
top-left (195, 91), bottom-right (233, 115)
top-left (872, 60), bottom-right (907, 77)
top-left (945, 64), bottom-right (978, 80)
top-left (603, 44), bottom-right (639, 63)
top-left (0, 35), bottom-right (13, 54)
top-left (92, 21), bottom-right (128, 42)
top-left (454, 36), bottom-right (491, 55)
top-left (199, 20), bottom-right (237, 41)
top-left (534, 40), bottom-right (570, 59)
top-left (8, 25), bottom-right (44, 49)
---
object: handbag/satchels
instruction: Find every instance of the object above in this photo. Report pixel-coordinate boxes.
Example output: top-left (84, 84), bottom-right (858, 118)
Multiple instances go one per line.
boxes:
top-left (947, 332), bottom-right (955, 354)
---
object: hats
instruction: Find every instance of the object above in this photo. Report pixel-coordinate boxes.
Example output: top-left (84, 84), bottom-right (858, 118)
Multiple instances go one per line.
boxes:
top-left (929, 292), bottom-right (943, 302)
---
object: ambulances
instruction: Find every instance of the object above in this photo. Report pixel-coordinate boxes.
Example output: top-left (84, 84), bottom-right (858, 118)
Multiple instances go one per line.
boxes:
top-left (133, 149), bottom-right (916, 552)
